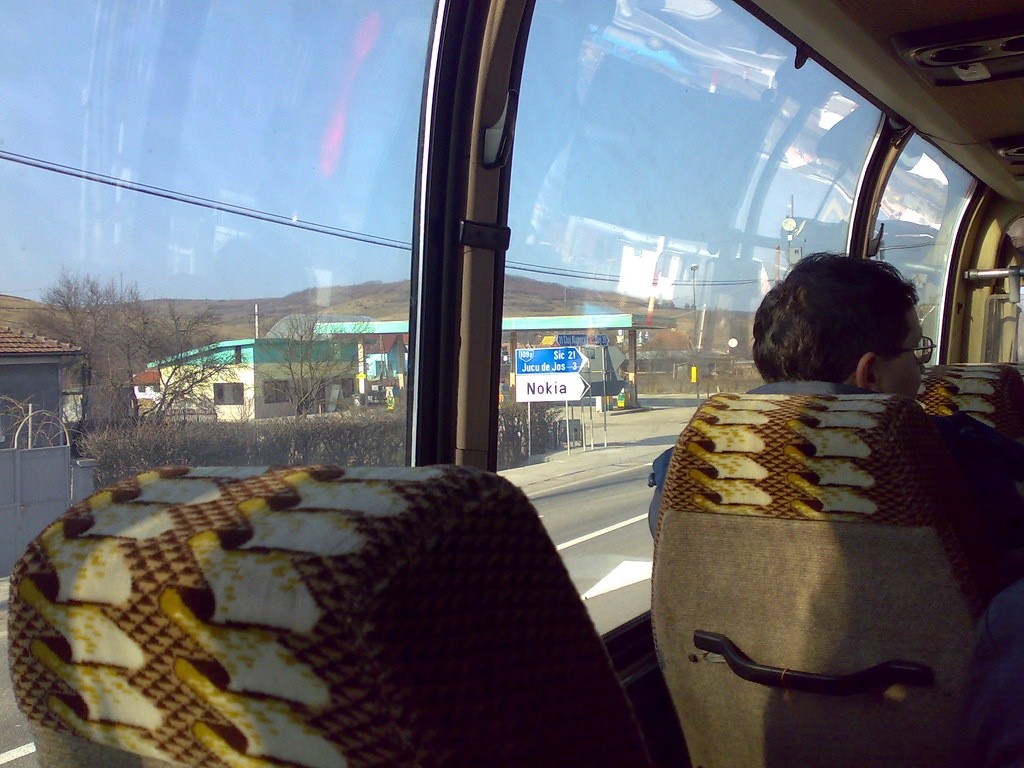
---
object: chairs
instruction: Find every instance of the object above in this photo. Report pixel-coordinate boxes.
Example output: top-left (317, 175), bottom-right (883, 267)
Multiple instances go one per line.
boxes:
top-left (915, 364), bottom-right (1023, 496)
top-left (6, 464), bottom-right (650, 768)
top-left (650, 391), bottom-right (999, 768)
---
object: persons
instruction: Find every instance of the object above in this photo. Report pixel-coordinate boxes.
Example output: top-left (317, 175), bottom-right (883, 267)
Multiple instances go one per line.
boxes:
top-left (648, 253), bottom-right (1024, 571)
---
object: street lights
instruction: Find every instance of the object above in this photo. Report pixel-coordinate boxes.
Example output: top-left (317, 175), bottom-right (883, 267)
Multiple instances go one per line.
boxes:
top-left (689, 263), bottom-right (700, 403)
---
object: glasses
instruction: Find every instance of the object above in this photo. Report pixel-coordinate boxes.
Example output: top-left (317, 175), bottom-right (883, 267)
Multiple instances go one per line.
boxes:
top-left (879, 336), bottom-right (934, 364)
